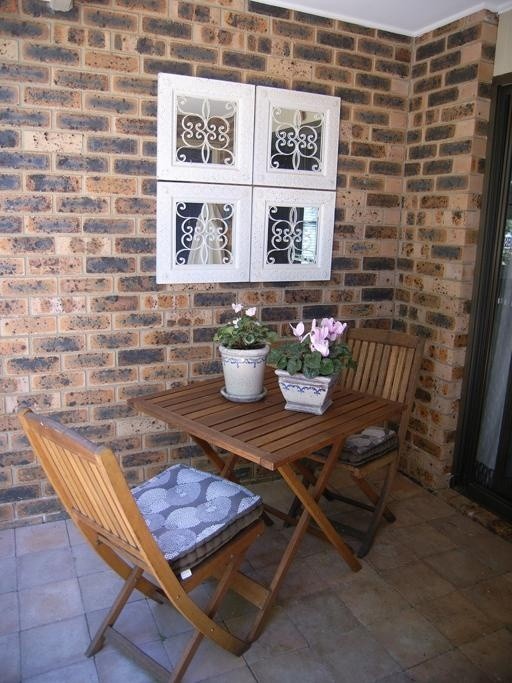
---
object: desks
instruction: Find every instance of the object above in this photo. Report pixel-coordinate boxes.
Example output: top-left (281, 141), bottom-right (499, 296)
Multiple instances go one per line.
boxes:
top-left (128, 365), bottom-right (407, 641)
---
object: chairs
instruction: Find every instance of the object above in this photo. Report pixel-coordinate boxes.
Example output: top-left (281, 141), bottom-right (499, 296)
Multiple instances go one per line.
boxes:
top-left (283, 327), bottom-right (425, 557)
top-left (18, 407), bottom-right (264, 683)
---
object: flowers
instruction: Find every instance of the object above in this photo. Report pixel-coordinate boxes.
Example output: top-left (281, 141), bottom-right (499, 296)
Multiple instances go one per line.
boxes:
top-left (213, 303), bottom-right (276, 347)
top-left (268, 317), bottom-right (358, 377)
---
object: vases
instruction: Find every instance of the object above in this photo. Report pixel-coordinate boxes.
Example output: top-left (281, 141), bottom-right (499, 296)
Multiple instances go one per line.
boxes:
top-left (218, 343), bottom-right (270, 402)
top-left (275, 368), bottom-right (333, 415)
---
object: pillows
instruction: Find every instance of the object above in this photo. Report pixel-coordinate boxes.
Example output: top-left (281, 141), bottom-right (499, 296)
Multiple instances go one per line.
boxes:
top-left (128, 463), bottom-right (263, 580)
top-left (319, 427), bottom-right (397, 465)
